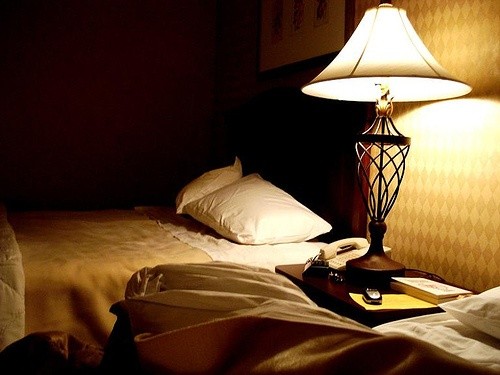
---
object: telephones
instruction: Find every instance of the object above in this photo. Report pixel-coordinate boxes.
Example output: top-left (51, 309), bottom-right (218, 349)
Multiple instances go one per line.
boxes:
top-left (307, 237), bottom-right (391, 273)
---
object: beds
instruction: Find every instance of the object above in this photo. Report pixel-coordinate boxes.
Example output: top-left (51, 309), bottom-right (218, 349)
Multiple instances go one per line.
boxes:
top-left (0, 198), bottom-right (337, 375)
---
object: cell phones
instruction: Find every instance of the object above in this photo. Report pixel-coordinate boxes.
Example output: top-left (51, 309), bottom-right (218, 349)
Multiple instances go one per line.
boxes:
top-left (364, 288), bottom-right (381, 304)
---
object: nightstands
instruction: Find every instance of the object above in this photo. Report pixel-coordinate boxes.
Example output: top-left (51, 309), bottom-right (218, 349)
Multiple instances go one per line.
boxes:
top-left (275, 262), bottom-right (457, 324)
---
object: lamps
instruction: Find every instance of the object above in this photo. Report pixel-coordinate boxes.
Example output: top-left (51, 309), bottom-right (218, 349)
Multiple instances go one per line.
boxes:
top-left (300, 2), bottom-right (473, 292)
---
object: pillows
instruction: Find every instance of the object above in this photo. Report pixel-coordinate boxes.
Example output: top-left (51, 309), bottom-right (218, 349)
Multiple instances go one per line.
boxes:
top-left (440, 288), bottom-right (499, 339)
top-left (176, 157), bottom-right (241, 215)
top-left (185, 172), bottom-right (335, 245)
